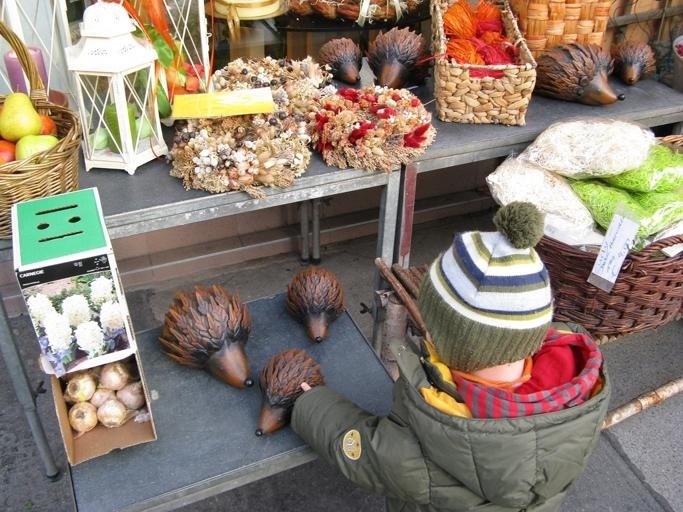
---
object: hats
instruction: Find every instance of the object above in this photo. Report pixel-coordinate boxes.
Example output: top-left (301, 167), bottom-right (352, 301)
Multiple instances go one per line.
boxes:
top-left (416, 202), bottom-right (555, 373)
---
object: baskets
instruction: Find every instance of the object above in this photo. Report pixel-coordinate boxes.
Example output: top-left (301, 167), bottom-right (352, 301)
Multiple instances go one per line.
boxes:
top-left (490, 134), bottom-right (683, 336)
top-left (430, 0), bottom-right (627, 126)
top-left (0, 17), bottom-right (82, 241)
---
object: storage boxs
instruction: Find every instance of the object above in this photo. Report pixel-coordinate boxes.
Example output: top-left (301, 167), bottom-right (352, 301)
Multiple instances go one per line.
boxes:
top-left (46, 338), bottom-right (156, 470)
top-left (7, 183), bottom-right (138, 376)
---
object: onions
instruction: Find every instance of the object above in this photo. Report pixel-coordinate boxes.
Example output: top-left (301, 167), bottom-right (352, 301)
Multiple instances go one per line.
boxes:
top-left (62, 362), bottom-right (143, 432)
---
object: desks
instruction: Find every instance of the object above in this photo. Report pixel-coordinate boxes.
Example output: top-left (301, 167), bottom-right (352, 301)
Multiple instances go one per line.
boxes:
top-left (1, 115), bottom-right (402, 485)
top-left (38, 284), bottom-right (393, 511)
top-left (396, 71), bottom-right (682, 272)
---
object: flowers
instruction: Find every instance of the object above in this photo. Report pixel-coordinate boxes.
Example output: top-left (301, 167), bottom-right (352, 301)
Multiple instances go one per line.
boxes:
top-left (23, 275), bottom-right (129, 375)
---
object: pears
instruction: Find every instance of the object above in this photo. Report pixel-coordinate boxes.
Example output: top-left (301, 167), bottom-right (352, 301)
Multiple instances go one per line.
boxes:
top-left (0, 80), bottom-right (60, 159)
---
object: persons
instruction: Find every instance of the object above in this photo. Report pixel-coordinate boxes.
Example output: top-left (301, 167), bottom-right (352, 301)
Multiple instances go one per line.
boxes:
top-left (289, 201), bottom-right (612, 511)
